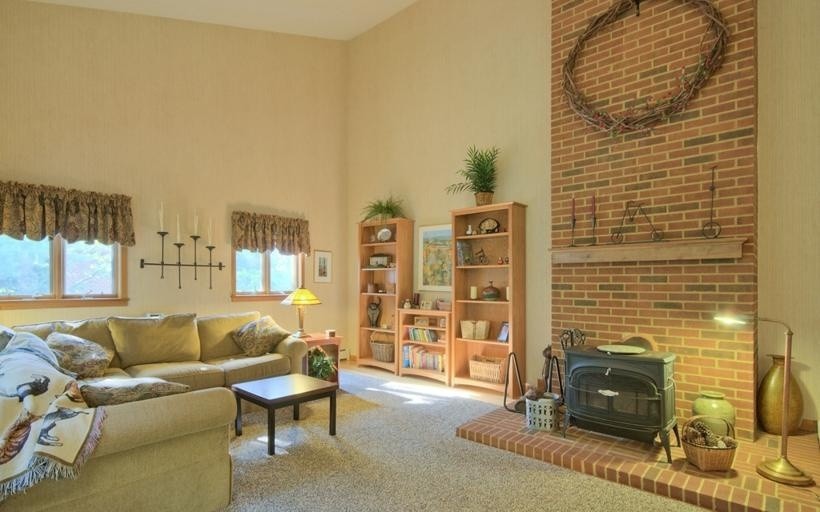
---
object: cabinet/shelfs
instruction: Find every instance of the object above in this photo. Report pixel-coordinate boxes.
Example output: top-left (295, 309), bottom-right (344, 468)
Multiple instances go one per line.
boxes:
top-left (395, 308), bottom-right (450, 386)
top-left (357, 218), bottom-right (414, 376)
top-left (450, 201), bottom-right (528, 400)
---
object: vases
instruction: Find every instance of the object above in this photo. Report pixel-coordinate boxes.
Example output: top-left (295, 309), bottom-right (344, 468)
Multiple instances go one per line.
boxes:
top-left (693, 390), bottom-right (736, 437)
top-left (756, 355), bottom-right (803, 437)
top-left (479, 279), bottom-right (500, 301)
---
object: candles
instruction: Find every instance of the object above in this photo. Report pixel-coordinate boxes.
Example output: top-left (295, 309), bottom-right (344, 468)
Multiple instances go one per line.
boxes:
top-left (159, 202), bottom-right (213, 246)
top-left (571, 195), bottom-right (596, 218)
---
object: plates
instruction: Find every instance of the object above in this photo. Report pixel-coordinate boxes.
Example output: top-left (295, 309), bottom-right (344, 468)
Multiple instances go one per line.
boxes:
top-left (376, 228), bottom-right (392, 242)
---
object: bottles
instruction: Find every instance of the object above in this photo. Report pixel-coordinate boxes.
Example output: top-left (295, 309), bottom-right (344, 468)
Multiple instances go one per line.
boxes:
top-left (403, 299), bottom-right (412, 308)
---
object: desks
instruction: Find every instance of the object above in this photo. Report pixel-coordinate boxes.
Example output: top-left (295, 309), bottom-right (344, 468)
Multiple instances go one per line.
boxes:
top-left (293, 332), bottom-right (343, 389)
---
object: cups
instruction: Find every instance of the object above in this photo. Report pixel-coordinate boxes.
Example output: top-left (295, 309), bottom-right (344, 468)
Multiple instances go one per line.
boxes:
top-left (367, 283), bottom-right (378, 293)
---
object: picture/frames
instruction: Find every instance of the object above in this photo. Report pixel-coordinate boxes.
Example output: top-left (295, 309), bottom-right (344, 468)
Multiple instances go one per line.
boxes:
top-left (496, 321), bottom-right (509, 342)
top-left (417, 222), bottom-right (450, 293)
top-left (313, 249), bottom-right (333, 284)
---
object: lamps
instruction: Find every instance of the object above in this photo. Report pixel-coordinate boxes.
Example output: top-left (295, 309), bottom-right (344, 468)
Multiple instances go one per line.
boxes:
top-left (712, 311), bottom-right (815, 488)
top-left (281, 284), bottom-right (322, 338)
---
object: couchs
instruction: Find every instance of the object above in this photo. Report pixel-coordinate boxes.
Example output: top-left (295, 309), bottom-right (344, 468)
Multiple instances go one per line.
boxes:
top-left (0, 311), bottom-right (307, 512)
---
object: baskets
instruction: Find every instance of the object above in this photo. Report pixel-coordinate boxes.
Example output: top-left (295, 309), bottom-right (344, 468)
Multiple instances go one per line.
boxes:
top-left (526, 392), bottom-right (560, 432)
top-left (369, 331), bottom-right (394, 362)
top-left (469, 354), bottom-right (508, 384)
top-left (681, 414), bottom-right (738, 472)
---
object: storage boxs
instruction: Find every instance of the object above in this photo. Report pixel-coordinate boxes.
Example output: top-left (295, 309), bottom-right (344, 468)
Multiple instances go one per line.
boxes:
top-left (368, 255), bottom-right (392, 268)
top-left (460, 319), bottom-right (491, 340)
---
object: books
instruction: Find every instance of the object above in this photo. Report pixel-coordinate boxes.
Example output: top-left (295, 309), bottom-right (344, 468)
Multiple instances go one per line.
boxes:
top-left (401, 327), bottom-right (447, 373)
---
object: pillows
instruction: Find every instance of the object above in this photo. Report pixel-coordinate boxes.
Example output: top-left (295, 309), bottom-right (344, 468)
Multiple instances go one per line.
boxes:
top-left (10, 310), bottom-right (292, 376)
top-left (77, 377), bottom-right (190, 407)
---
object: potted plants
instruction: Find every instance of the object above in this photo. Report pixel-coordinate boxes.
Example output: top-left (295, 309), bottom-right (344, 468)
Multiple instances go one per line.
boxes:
top-left (444, 142), bottom-right (501, 207)
top-left (360, 195), bottom-right (408, 227)
